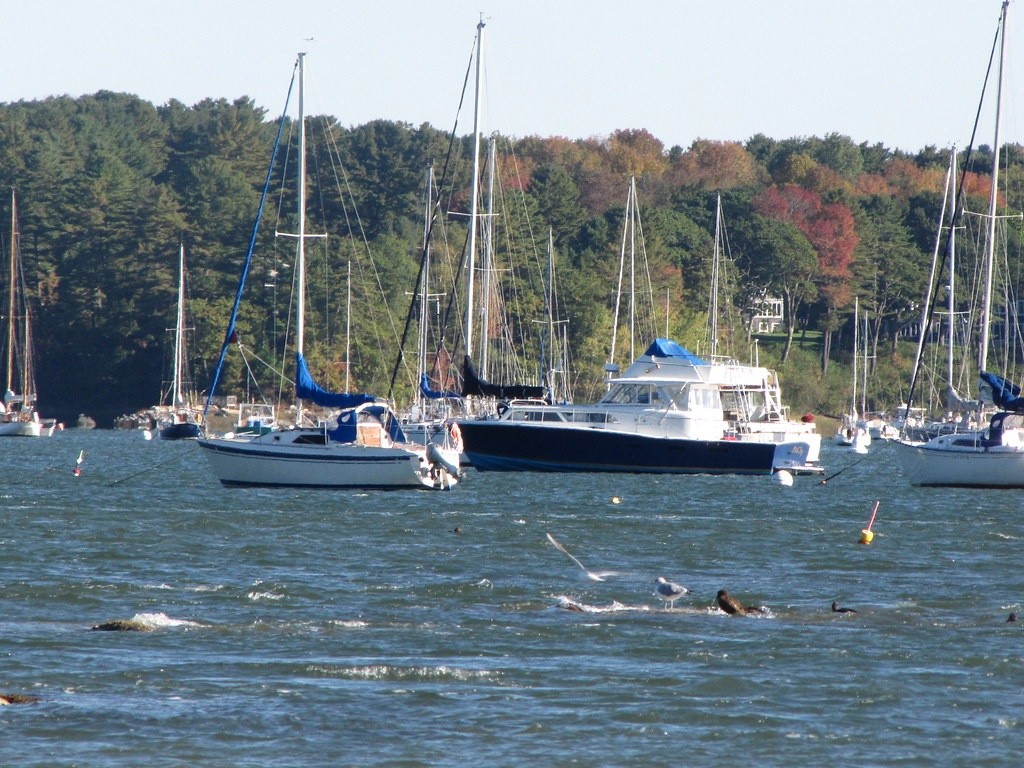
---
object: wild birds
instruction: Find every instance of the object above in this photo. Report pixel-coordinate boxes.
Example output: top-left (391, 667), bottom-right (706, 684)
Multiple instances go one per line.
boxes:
top-left (651, 577), bottom-right (695, 613)
top-left (545, 532), bottom-right (621, 586)
top-left (1006, 612), bottom-right (1019, 623)
top-left (832, 601), bottom-right (858, 613)
top-left (712, 589), bottom-right (764, 617)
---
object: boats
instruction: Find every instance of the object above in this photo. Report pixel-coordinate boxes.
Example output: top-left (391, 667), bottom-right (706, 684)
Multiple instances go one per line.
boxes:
top-left (0, 3), bottom-right (1024, 495)
